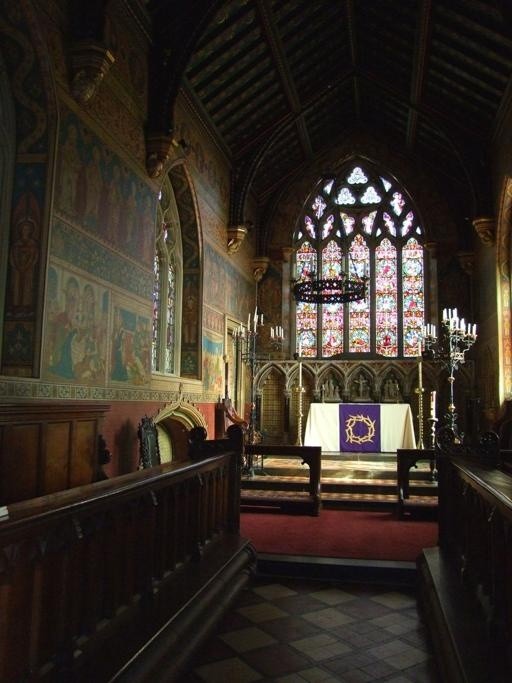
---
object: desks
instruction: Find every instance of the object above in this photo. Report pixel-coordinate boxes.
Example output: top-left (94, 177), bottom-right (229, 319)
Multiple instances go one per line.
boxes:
top-left (301, 400), bottom-right (419, 452)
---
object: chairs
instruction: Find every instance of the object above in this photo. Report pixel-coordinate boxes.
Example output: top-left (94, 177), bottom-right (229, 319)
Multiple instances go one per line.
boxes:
top-left (136, 411), bottom-right (163, 469)
top-left (219, 392), bottom-right (265, 475)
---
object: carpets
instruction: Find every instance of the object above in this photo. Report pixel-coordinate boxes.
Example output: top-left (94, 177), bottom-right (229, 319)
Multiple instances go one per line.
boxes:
top-left (238, 494), bottom-right (438, 559)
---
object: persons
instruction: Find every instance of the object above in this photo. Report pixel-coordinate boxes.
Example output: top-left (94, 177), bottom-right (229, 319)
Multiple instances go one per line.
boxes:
top-left (56, 122), bottom-right (153, 260)
top-left (7, 329), bottom-right (34, 368)
top-left (50, 311), bottom-right (152, 385)
top-left (8, 216), bottom-right (41, 316)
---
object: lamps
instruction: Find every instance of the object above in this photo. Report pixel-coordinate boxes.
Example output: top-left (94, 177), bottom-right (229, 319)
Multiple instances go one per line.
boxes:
top-left (289, 142), bottom-right (368, 306)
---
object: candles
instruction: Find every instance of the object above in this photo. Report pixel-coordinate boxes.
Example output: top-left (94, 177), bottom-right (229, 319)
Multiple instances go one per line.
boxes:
top-left (417, 361), bottom-right (424, 388)
top-left (419, 305), bottom-right (478, 340)
top-left (231, 303), bottom-right (285, 340)
top-left (299, 360), bottom-right (302, 386)
top-left (430, 389), bottom-right (437, 416)
